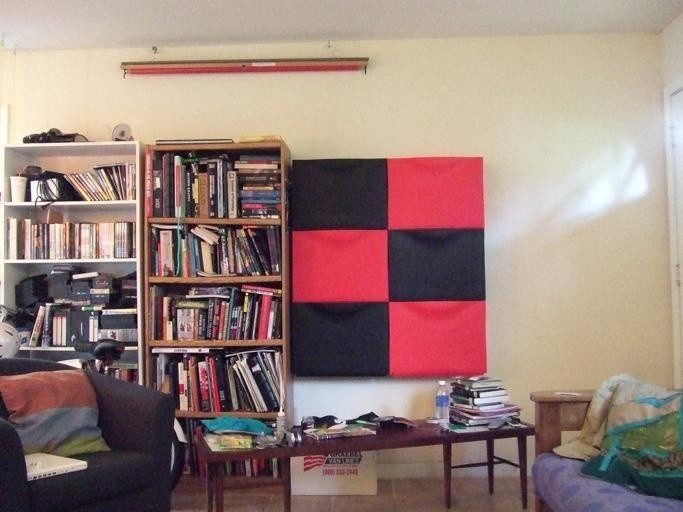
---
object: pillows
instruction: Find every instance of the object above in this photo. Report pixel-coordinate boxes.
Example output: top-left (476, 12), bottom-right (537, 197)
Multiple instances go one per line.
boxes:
top-left (0, 369), bottom-right (96, 460)
top-left (553, 373), bottom-right (683, 463)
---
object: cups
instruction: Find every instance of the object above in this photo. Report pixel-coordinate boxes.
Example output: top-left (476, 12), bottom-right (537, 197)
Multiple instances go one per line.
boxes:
top-left (8, 176), bottom-right (28, 202)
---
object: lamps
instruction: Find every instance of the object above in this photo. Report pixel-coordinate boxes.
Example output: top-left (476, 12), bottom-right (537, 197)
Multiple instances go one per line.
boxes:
top-left (121, 57), bottom-right (369, 79)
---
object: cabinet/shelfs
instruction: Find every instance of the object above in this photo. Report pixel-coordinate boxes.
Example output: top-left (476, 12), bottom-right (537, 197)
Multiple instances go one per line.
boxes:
top-left (145, 139), bottom-right (291, 485)
top-left (0, 141), bottom-right (144, 386)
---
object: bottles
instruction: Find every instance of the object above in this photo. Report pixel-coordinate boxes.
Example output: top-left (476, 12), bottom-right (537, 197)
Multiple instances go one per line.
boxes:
top-left (435, 380), bottom-right (450, 423)
top-left (276, 404), bottom-right (287, 434)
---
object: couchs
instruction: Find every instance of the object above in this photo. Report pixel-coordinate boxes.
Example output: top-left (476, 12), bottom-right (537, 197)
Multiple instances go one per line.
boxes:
top-left (530, 390), bottom-right (683, 512)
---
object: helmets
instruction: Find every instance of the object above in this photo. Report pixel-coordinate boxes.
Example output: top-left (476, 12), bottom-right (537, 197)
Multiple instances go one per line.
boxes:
top-left (0, 323), bottom-right (20, 359)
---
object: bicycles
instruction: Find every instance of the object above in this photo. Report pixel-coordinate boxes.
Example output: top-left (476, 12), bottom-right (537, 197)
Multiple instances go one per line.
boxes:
top-left (72, 339), bottom-right (188, 492)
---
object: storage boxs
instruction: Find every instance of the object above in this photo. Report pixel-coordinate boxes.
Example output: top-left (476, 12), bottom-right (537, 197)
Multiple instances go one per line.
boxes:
top-left (292, 449), bottom-right (377, 497)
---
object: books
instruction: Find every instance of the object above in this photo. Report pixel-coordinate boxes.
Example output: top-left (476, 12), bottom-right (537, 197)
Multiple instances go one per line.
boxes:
top-left (146, 151), bottom-right (281, 481)
top-left (237, 134), bottom-right (291, 156)
top-left (25, 454), bottom-right (88, 479)
top-left (451, 376), bottom-right (520, 424)
top-left (156, 139), bottom-right (234, 144)
top-left (11, 162), bottom-right (138, 384)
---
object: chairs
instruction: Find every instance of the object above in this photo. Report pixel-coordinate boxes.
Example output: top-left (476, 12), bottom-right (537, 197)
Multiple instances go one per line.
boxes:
top-left (0, 358), bottom-right (175, 512)
